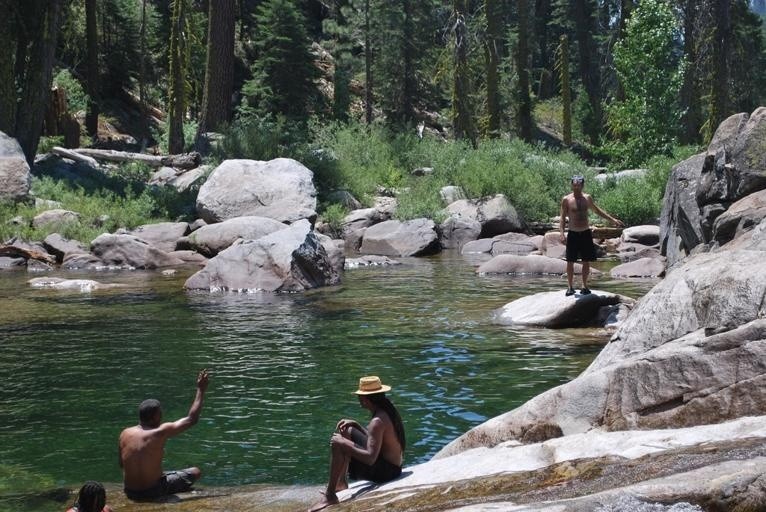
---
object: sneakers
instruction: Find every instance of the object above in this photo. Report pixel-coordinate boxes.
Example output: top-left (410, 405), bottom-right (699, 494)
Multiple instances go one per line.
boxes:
top-left (565, 286), bottom-right (592, 297)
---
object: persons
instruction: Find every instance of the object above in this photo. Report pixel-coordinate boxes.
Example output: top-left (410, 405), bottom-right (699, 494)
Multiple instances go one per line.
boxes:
top-left (68, 478), bottom-right (112, 512)
top-left (307, 376), bottom-right (406, 512)
top-left (560, 175), bottom-right (625, 296)
top-left (118, 368), bottom-right (208, 502)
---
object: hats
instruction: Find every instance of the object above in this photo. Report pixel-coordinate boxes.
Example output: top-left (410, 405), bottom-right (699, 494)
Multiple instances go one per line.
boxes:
top-left (350, 376), bottom-right (392, 396)
